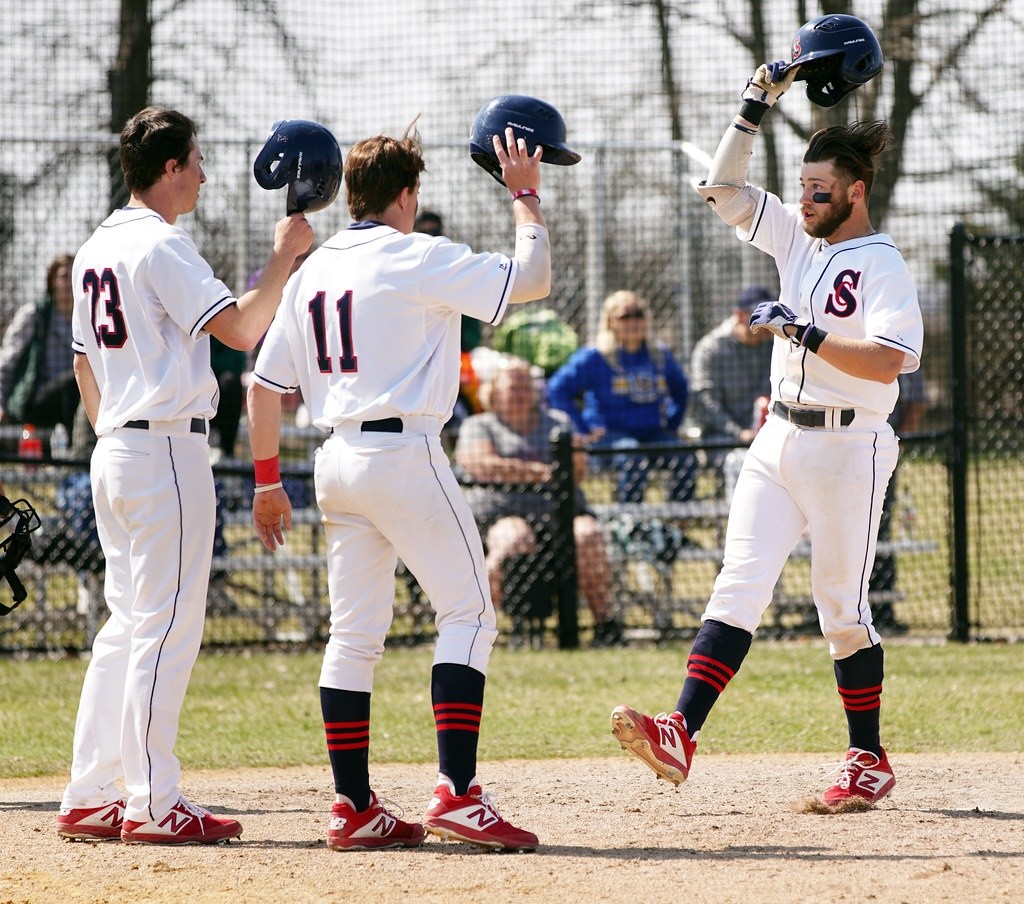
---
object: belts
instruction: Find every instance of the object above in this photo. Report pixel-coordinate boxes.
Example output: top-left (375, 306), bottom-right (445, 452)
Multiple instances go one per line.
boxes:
top-left (772, 400), bottom-right (854, 428)
top-left (122, 417), bottom-right (206, 433)
top-left (330, 417), bottom-right (403, 433)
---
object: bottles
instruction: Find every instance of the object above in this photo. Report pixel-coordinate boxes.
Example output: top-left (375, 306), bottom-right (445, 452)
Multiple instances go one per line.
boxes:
top-left (50, 423), bottom-right (69, 460)
top-left (17, 423), bottom-right (43, 460)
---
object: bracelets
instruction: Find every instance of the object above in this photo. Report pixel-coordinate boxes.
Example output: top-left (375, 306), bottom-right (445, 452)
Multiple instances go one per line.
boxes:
top-left (804, 326), bottom-right (828, 353)
top-left (255, 481), bottom-right (283, 493)
top-left (738, 99), bottom-right (769, 126)
top-left (512, 188), bottom-right (541, 204)
top-left (254, 455), bottom-right (280, 484)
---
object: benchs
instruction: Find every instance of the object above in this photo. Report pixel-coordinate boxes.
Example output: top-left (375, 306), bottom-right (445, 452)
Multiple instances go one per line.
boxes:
top-left (15, 498), bottom-right (942, 643)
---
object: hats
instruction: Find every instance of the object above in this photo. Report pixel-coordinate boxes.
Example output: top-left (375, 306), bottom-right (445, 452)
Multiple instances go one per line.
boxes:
top-left (736, 287), bottom-right (772, 318)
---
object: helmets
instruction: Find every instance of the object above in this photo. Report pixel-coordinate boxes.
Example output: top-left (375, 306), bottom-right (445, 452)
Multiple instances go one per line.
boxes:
top-left (468, 95), bottom-right (581, 188)
top-left (783, 13), bottom-right (883, 109)
top-left (253, 118), bottom-right (343, 216)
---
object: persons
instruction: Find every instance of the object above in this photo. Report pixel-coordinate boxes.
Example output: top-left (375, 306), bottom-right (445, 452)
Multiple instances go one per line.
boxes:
top-left (546, 292), bottom-right (699, 560)
top-left (868, 372), bottom-right (926, 633)
top-left (1, 254), bottom-right (245, 613)
top-left (456, 363), bottom-right (627, 646)
top-left (414, 210), bottom-right (481, 424)
top-left (611, 62), bottom-right (924, 803)
top-left (57, 108), bottom-right (315, 845)
top-left (689, 287), bottom-right (776, 468)
top-left (248, 127), bottom-right (552, 851)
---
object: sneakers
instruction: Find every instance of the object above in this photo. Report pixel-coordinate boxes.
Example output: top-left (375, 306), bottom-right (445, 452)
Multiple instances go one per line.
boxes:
top-left (422, 784), bottom-right (539, 853)
top-left (822, 747), bottom-right (896, 807)
top-left (326, 790), bottom-right (427, 851)
top-left (121, 793), bottom-right (243, 845)
top-left (56, 799), bottom-right (129, 842)
top-left (610, 704), bottom-right (698, 786)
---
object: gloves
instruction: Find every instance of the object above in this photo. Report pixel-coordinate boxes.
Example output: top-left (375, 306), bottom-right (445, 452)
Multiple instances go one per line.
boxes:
top-left (749, 301), bottom-right (816, 347)
top-left (742, 62), bottom-right (800, 107)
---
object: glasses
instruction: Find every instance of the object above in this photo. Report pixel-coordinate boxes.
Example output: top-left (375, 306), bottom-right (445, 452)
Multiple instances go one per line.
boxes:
top-left (613, 309), bottom-right (644, 322)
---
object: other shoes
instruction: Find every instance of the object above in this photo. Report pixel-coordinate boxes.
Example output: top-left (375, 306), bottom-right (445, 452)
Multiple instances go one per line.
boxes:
top-left (869, 607), bottom-right (908, 637)
top-left (595, 623), bottom-right (630, 647)
